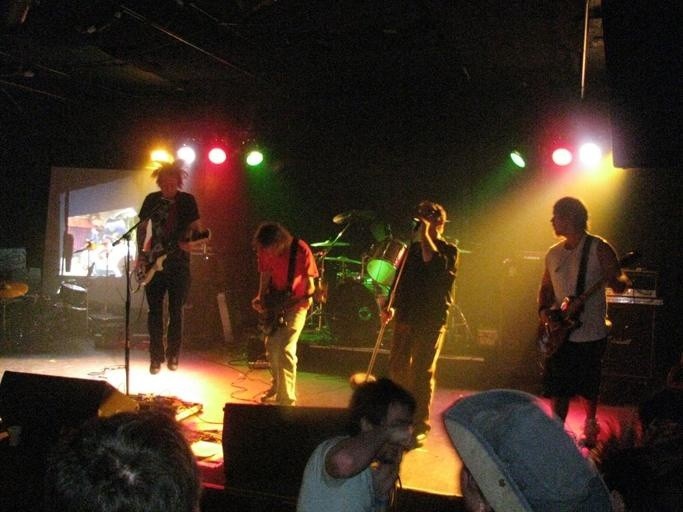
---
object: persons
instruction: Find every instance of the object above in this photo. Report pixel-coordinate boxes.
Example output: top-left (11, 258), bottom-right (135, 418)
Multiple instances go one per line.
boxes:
top-left (379, 201), bottom-right (459, 444)
top-left (460, 359), bottom-right (683, 511)
top-left (250, 222), bottom-right (319, 408)
top-left (40, 406), bottom-right (203, 512)
top-left (537, 198), bottom-right (632, 449)
top-left (136, 160), bottom-right (206, 374)
top-left (295, 375), bottom-right (417, 512)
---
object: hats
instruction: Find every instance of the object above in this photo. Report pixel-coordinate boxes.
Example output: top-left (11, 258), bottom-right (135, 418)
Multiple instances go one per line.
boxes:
top-left (442, 389), bottom-right (614, 511)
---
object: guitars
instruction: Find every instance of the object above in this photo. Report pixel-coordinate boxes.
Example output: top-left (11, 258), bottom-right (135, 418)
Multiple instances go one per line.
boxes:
top-left (257, 288), bottom-right (325, 336)
top-left (134, 227), bottom-right (211, 286)
top-left (536, 252), bottom-right (636, 358)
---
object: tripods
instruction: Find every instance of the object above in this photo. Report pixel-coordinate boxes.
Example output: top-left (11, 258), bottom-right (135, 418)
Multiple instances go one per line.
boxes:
top-left (279, 256), bottom-right (306, 331)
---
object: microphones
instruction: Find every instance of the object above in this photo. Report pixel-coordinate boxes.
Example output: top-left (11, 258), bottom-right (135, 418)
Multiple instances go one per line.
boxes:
top-left (413, 217), bottom-right (421, 226)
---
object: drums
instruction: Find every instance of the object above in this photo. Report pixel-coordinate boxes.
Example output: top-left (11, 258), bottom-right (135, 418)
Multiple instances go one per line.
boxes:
top-left (367, 238), bottom-right (407, 286)
top-left (61, 282), bottom-right (86, 306)
top-left (328, 277), bottom-right (391, 342)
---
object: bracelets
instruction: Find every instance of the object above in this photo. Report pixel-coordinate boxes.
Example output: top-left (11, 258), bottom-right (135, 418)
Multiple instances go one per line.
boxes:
top-left (538, 304), bottom-right (547, 319)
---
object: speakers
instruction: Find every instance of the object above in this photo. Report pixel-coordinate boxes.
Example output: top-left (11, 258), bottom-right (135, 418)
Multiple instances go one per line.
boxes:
top-left (599, 1), bottom-right (683, 169)
top-left (221, 403), bottom-right (350, 511)
top-left (601, 302), bottom-right (662, 407)
top-left (0, 370), bottom-right (142, 420)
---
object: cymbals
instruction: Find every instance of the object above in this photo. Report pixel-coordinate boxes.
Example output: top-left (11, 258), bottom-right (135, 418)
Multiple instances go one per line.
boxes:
top-left (323, 256), bottom-right (362, 264)
top-left (457, 248), bottom-right (470, 254)
top-left (0, 282), bottom-right (30, 299)
top-left (311, 241), bottom-right (350, 247)
top-left (333, 209), bottom-right (375, 224)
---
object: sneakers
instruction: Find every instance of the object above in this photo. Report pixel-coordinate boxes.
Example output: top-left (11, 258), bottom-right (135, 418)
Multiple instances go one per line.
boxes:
top-left (150, 359), bottom-right (160, 374)
top-left (412, 430), bottom-right (426, 448)
top-left (168, 357), bottom-right (178, 369)
